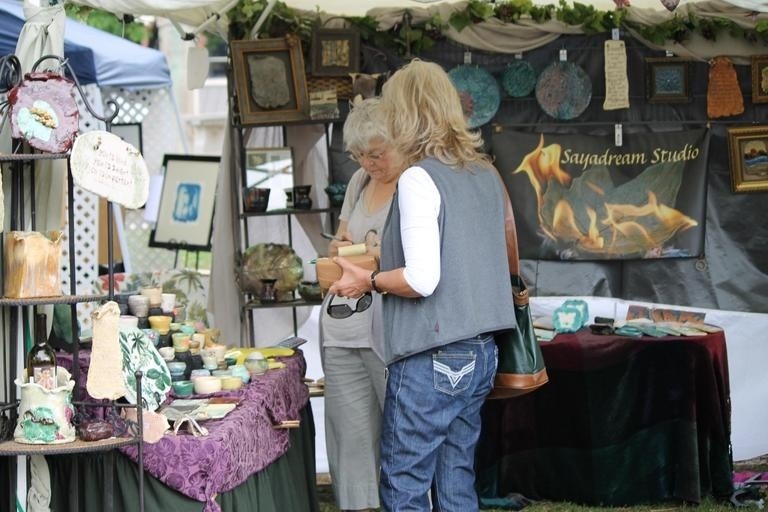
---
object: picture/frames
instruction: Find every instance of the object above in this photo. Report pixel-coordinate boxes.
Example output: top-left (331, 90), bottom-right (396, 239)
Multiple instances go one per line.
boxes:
top-left (728, 126), bottom-right (768, 194)
top-left (232, 36), bottom-right (309, 124)
top-left (311, 26), bottom-right (361, 78)
top-left (644, 57), bottom-right (696, 104)
top-left (750, 53), bottom-right (768, 104)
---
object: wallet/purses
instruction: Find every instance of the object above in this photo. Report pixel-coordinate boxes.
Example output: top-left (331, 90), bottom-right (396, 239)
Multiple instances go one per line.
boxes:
top-left (316, 254), bottom-right (381, 292)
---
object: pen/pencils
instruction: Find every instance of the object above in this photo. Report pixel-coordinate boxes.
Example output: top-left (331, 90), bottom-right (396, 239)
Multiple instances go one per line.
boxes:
top-left (320, 232), bottom-right (341, 242)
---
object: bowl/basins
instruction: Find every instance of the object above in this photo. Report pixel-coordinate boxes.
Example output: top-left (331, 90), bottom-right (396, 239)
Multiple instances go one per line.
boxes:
top-left (172, 380), bottom-right (193, 397)
top-left (147, 316), bottom-right (173, 335)
top-left (140, 287), bottom-right (161, 308)
top-left (194, 376), bottom-right (220, 394)
top-left (167, 361), bottom-right (187, 376)
top-left (160, 346), bottom-right (174, 361)
top-left (221, 376), bottom-right (242, 389)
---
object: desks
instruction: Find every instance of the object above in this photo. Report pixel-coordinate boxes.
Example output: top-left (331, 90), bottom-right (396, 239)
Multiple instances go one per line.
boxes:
top-left (54, 348), bottom-right (316, 511)
top-left (488, 318), bottom-right (731, 504)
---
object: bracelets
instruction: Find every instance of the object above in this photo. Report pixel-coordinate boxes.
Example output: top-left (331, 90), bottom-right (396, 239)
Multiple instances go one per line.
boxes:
top-left (370, 270), bottom-right (390, 296)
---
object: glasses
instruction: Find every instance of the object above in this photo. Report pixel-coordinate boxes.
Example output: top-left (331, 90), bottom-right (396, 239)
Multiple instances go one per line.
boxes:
top-left (350, 142), bottom-right (393, 162)
top-left (326, 291), bottom-right (373, 319)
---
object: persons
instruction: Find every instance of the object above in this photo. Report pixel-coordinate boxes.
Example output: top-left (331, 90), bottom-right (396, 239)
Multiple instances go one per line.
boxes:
top-left (317, 97), bottom-right (414, 512)
top-left (328, 61), bottom-right (516, 512)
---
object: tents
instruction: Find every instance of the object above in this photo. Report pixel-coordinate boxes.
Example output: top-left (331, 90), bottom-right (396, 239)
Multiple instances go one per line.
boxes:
top-left (0, 0), bottom-right (190, 290)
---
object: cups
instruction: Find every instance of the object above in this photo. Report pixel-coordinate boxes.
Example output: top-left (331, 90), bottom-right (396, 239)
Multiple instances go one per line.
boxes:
top-left (171, 333), bottom-right (190, 352)
top-left (191, 333), bottom-right (205, 349)
top-left (160, 294), bottom-right (176, 314)
top-left (128, 295), bottom-right (150, 318)
top-left (199, 349), bottom-right (218, 370)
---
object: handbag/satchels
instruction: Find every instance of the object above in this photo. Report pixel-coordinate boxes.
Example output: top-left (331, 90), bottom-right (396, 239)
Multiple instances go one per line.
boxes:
top-left (479, 154), bottom-right (548, 401)
